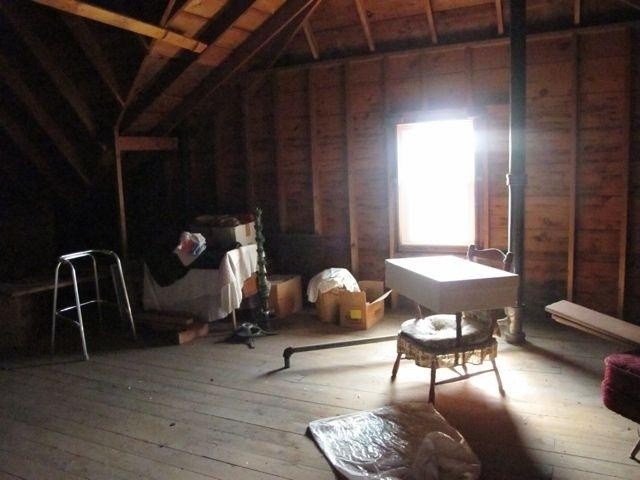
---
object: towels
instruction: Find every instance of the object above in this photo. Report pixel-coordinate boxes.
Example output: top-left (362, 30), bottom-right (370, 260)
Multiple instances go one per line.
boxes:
top-left (305, 268), bottom-right (360, 304)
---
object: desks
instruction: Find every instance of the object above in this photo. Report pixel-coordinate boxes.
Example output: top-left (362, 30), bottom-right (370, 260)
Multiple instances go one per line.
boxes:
top-left (384, 253), bottom-right (520, 366)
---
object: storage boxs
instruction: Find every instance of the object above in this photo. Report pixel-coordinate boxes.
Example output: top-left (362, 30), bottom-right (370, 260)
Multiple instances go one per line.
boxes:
top-left (266, 273), bottom-right (392, 330)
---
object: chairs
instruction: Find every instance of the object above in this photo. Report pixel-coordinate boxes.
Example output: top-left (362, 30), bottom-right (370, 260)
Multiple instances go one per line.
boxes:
top-left (390, 244), bottom-right (514, 403)
